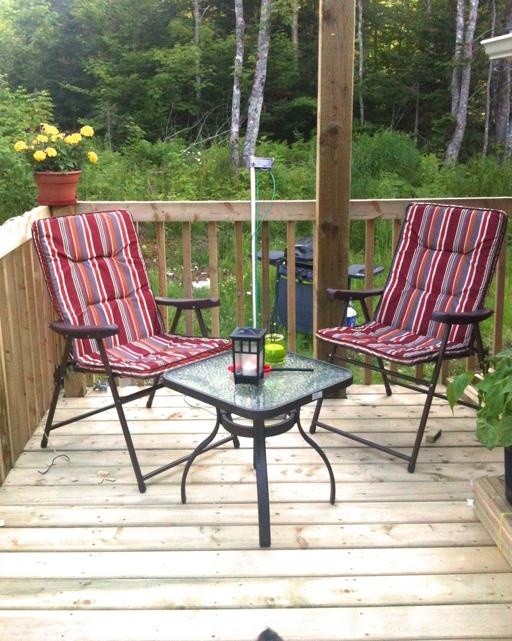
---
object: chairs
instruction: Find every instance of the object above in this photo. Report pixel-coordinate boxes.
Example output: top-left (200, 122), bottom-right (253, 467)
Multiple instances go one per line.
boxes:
top-left (309, 201), bottom-right (509, 474)
top-left (29, 209), bottom-right (240, 492)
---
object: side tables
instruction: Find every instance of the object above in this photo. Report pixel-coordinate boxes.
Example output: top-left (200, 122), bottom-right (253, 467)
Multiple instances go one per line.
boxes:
top-left (162, 346), bottom-right (354, 548)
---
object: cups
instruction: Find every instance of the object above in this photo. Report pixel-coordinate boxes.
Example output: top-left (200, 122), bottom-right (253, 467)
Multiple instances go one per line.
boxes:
top-left (265, 333), bottom-right (286, 365)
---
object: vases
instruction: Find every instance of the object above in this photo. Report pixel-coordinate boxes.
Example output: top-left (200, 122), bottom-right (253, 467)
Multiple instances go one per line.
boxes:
top-left (33, 170), bottom-right (82, 205)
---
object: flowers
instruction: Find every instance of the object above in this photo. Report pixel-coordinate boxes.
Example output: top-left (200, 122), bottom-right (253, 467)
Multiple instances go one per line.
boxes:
top-left (12, 122), bottom-right (98, 170)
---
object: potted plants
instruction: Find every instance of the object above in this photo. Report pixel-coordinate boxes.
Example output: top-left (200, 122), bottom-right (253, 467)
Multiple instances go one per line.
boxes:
top-left (446, 348), bottom-right (512, 505)
